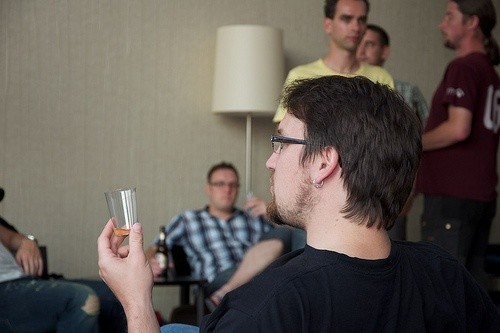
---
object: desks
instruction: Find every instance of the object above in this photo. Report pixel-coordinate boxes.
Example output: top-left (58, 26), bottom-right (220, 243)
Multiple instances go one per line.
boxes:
top-left (152, 269), bottom-right (208, 328)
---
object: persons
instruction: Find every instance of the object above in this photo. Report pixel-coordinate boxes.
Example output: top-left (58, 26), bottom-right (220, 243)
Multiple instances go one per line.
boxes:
top-left (96, 76), bottom-right (500, 333)
top-left (272, 0), bottom-right (500, 242)
top-left (144, 161), bottom-right (294, 312)
top-left (0, 190), bottom-right (100, 333)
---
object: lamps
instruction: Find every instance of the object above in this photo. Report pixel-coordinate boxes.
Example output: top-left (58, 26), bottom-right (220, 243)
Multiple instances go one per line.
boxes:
top-left (212, 24), bottom-right (286, 198)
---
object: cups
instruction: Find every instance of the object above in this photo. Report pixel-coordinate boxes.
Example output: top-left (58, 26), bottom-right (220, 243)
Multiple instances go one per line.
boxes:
top-left (104, 187), bottom-right (140, 236)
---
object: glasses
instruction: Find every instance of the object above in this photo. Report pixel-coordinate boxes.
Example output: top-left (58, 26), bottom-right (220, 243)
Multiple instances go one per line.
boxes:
top-left (210, 182), bottom-right (240, 188)
top-left (271, 134), bottom-right (307, 154)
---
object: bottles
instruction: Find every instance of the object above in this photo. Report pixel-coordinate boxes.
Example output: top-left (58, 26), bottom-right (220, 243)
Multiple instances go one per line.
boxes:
top-left (155, 225), bottom-right (169, 279)
